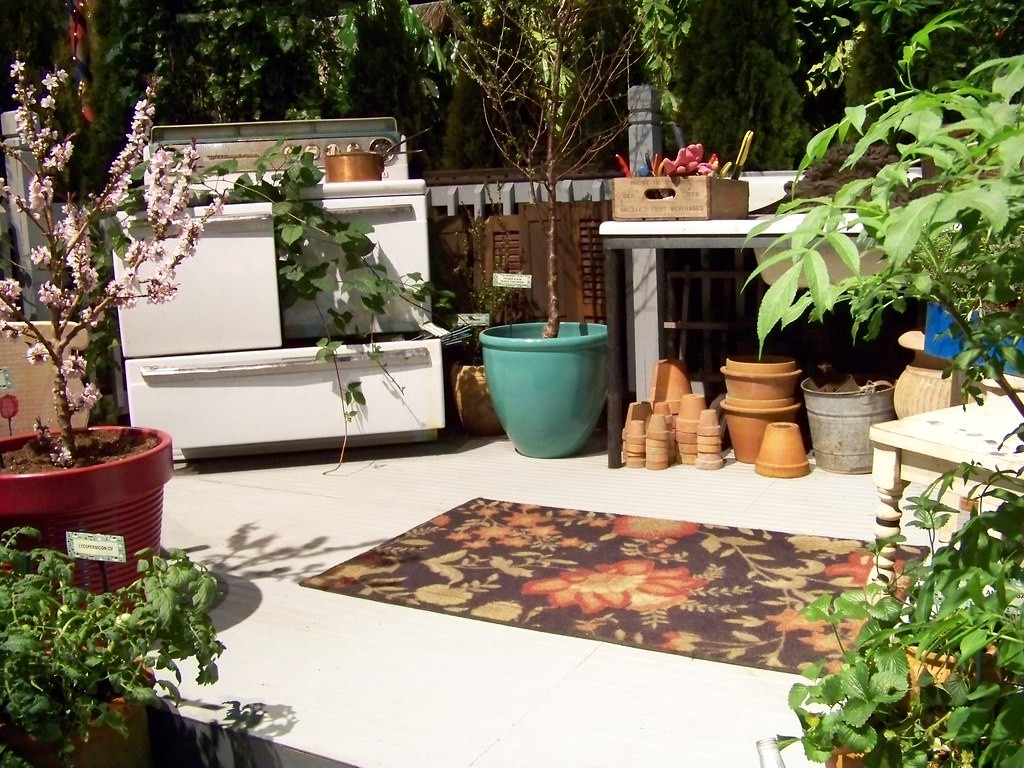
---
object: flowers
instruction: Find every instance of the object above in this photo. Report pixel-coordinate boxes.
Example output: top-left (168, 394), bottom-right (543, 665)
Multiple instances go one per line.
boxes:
top-left (0, 50), bottom-right (231, 465)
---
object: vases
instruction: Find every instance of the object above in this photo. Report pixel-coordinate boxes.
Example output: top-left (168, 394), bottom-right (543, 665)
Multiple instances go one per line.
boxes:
top-left (0, 426), bottom-right (172, 647)
top-left (621, 324), bottom-right (969, 480)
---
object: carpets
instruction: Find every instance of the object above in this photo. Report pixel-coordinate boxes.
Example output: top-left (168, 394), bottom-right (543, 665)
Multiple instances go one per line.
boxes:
top-left (299, 496), bottom-right (932, 678)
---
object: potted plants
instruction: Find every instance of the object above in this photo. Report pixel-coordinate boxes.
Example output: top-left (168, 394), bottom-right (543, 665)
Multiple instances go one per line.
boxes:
top-left (792, 424), bottom-right (1024, 768)
top-left (423, 0), bottom-right (693, 459)
top-left (0, 525), bottom-right (226, 768)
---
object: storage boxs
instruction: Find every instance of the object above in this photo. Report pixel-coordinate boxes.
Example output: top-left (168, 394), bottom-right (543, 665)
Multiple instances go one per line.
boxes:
top-left (613, 175), bottom-right (750, 220)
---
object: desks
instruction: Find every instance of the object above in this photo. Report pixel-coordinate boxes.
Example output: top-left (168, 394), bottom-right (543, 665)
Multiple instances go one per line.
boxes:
top-left (866, 399), bottom-right (1024, 607)
top-left (596, 209), bottom-right (962, 470)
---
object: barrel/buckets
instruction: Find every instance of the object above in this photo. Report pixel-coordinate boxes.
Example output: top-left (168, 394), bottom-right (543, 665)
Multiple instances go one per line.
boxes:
top-left (622, 354), bottom-right (898, 479)
top-left (324, 152), bottom-right (383, 182)
